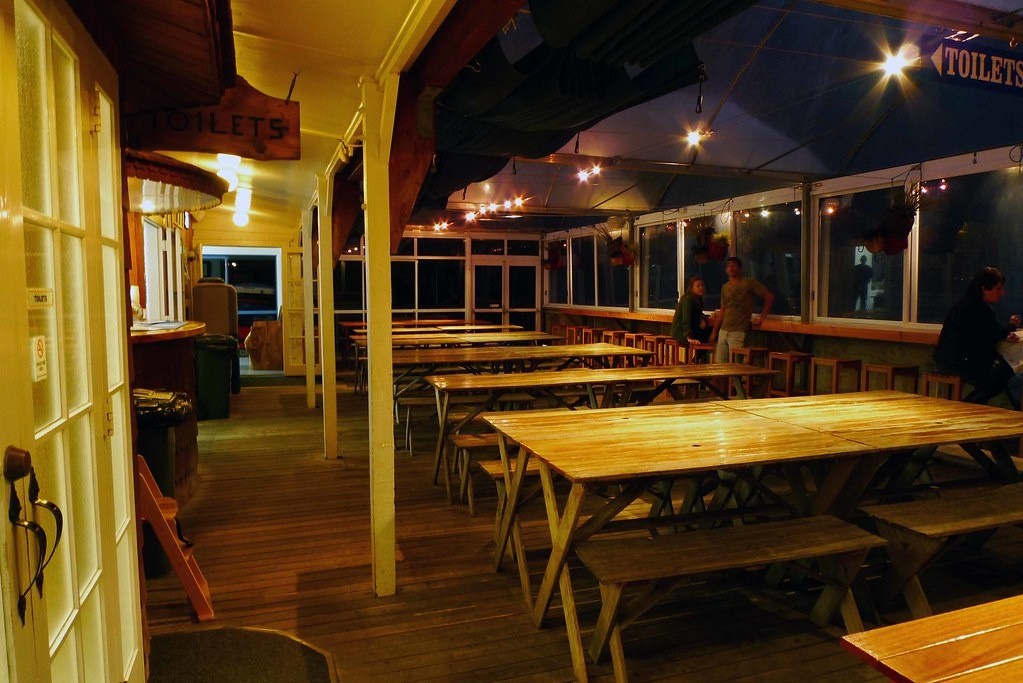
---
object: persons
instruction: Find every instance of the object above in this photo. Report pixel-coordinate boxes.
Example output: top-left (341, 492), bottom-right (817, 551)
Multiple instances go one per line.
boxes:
top-left (932, 266), bottom-right (1014, 414)
top-left (709, 257), bottom-right (774, 394)
top-left (669, 277), bottom-right (712, 363)
top-left (1006, 330), bottom-right (1023, 411)
top-left (853, 255), bottom-right (873, 309)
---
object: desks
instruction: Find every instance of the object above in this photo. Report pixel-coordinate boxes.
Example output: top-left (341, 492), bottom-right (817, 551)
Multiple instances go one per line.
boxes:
top-left (482, 389), bottom-right (1023, 683)
top-left (426, 363), bottom-right (783, 506)
top-left (339, 320), bottom-right (657, 456)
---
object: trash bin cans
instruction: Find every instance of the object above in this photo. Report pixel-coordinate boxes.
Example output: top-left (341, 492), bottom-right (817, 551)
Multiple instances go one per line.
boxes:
top-left (195, 334), bottom-right (240, 420)
top-left (133, 388), bottom-right (191, 576)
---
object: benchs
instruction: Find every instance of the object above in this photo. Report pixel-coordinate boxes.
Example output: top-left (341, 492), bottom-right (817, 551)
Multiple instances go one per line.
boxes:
top-left (855, 482), bottom-right (1023, 627)
top-left (547, 378), bottom-right (700, 408)
top-left (841, 595), bottom-right (1023, 683)
top-left (449, 433), bottom-right (519, 515)
top-left (393, 376), bottom-right (431, 391)
top-left (481, 360), bottom-right (576, 369)
top-left (625, 395), bottom-right (752, 407)
top-left (573, 515), bottom-right (890, 683)
top-left (398, 392), bottom-right (535, 458)
top-left (478, 459), bottom-right (544, 561)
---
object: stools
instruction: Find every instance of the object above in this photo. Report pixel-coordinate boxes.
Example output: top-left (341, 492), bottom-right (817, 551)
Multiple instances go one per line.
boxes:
top-left (602, 329), bottom-right (629, 369)
top-left (565, 325), bottom-right (592, 345)
top-left (664, 338), bottom-right (689, 396)
top-left (921, 369), bottom-right (964, 402)
top-left (623, 332), bottom-right (654, 368)
top-left (728, 346), bottom-right (769, 398)
top-left (581, 327), bottom-right (607, 369)
top-left (687, 343), bottom-right (718, 396)
top-left (643, 334), bottom-right (671, 367)
top-left (550, 324), bottom-right (567, 345)
top-left (767, 349), bottom-right (813, 399)
top-left (809, 356), bottom-right (862, 396)
top-left (862, 362), bottom-right (919, 394)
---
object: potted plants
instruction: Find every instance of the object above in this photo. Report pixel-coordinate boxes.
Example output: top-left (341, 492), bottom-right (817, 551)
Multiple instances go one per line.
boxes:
top-left (541, 246), bottom-right (563, 270)
top-left (688, 210), bottom-right (732, 266)
top-left (589, 221), bottom-right (637, 265)
top-left (856, 179), bottom-right (924, 254)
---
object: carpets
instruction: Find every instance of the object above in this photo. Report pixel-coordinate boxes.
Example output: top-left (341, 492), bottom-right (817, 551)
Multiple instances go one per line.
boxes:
top-left (149, 623), bottom-right (338, 683)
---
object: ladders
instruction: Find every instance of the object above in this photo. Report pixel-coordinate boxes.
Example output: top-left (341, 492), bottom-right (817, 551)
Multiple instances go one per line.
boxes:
top-left (135, 454), bottom-right (214, 623)
